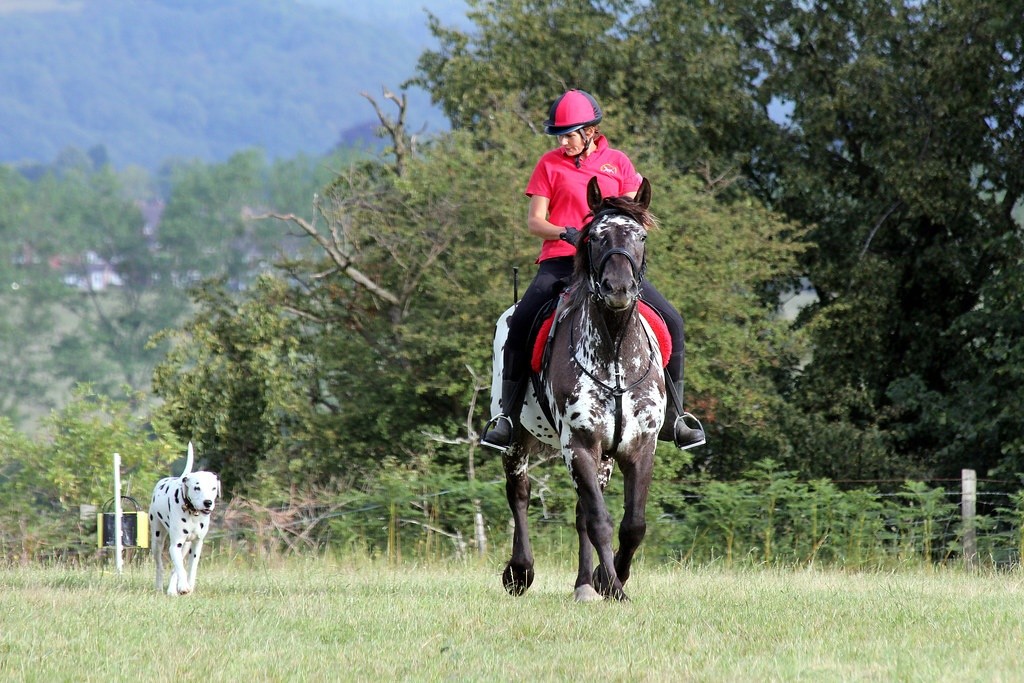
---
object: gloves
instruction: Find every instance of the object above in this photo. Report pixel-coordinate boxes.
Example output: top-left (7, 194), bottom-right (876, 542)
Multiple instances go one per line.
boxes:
top-left (559, 226), bottom-right (581, 248)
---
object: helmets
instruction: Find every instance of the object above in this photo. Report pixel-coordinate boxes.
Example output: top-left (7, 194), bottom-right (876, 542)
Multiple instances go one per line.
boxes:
top-left (543, 88), bottom-right (602, 136)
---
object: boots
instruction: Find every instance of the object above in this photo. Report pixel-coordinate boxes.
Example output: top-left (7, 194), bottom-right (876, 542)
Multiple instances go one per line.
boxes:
top-left (657, 344), bottom-right (703, 443)
top-left (485, 380), bottom-right (527, 445)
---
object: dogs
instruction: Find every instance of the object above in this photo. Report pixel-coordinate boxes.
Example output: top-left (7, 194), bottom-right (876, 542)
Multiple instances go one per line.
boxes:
top-left (149, 440), bottom-right (221, 597)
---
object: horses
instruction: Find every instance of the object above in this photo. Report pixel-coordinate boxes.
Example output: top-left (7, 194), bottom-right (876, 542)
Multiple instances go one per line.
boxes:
top-left (488, 176), bottom-right (675, 603)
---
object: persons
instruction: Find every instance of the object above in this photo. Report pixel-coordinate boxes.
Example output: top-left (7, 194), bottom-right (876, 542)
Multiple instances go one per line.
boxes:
top-left (481, 83), bottom-right (707, 455)
